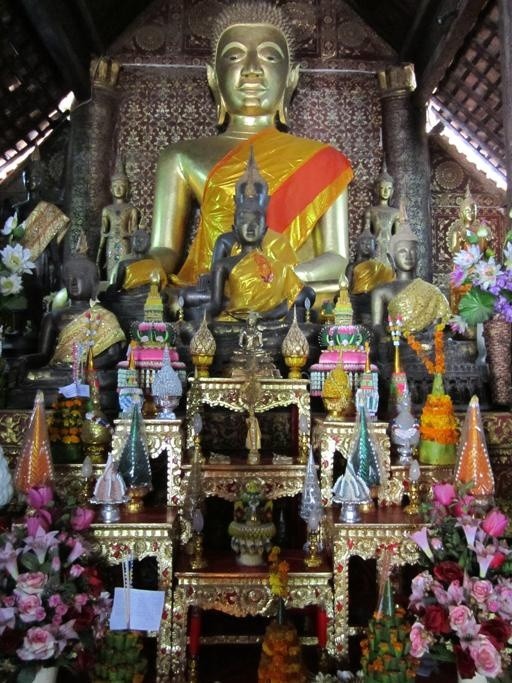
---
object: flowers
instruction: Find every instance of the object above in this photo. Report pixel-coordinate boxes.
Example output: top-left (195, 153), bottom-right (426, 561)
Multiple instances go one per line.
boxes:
top-left (0, 482), bottom-right (114, 679)
top-left (444, 216), bottom-right (512, 335)
top-left (0, 211), bottom-right (37, 333)
top-left (408, 478), bottom-right (512, 683)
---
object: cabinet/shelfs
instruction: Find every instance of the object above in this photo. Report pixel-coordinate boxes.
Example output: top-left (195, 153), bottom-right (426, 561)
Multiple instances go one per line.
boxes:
top-left (10, 377), bottom-right (457, 683)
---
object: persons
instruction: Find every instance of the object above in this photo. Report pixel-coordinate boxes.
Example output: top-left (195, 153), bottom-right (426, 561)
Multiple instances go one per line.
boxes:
top-left (181, 172), bottom-right (316, 348)
top-left (11, 258), bottom-right (120, 373)
top-left (344, 231), bottom-right (450, 341)
top-left (12, 164), bottom-right (62, 299)
top-left (93, 173), bottom-right (167, 301)
top-left (363, 172), bottom-right (401, 236)
top-left (149, 0), bottom-right (349, 295)
top-left (450, 198), bottom-right (487, 252)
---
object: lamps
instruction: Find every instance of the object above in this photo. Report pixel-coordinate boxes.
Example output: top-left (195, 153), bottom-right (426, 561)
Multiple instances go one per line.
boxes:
top-left (304, 297), bottom-right (312, 324)
top-left (178, 296), bottom-right (185, 322)
